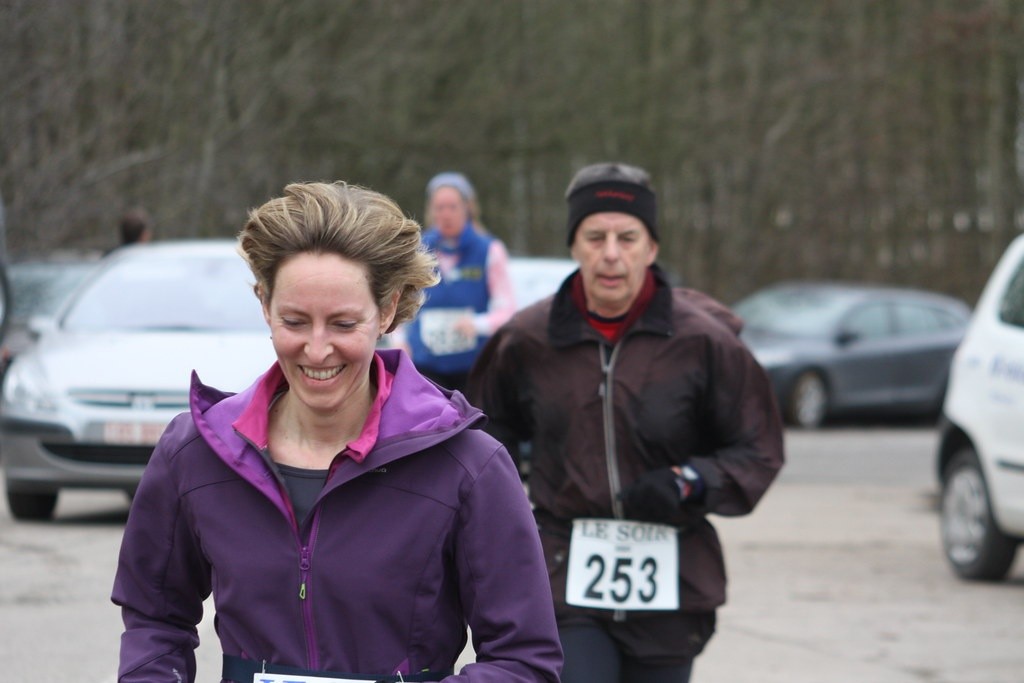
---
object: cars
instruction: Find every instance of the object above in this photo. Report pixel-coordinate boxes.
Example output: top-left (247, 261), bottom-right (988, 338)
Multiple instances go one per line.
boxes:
top-left (723, 281), bottom-right (978, 429)
top-left (936, 231), bottom-right (1024, 584)
top-left (505, 254), bottom-right (581, 326)
top-left (0, 261), bottom-right (94, 364)
top-left (0, 239), bottom-right (289, 519)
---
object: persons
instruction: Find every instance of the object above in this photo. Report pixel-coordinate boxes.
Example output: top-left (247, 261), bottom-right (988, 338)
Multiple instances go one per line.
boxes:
top-left (116, 204), bottom-right (154, 247)
top-left (393, 169), bottom-right (518, 397)
top-left (459, 159), bottom-right (786, 683)
top-left (109, 180), bottom-right (565, 683)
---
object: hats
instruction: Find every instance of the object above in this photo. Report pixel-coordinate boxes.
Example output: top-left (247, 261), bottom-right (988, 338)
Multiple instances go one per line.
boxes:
top-left (566, 162), bottom-right (660, 245)
top-left (426, 174), bottom-right (473, 211)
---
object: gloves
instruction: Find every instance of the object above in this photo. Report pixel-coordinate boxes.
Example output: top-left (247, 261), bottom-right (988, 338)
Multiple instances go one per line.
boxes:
top-left (628, 464), bottom-right (703, 520)
